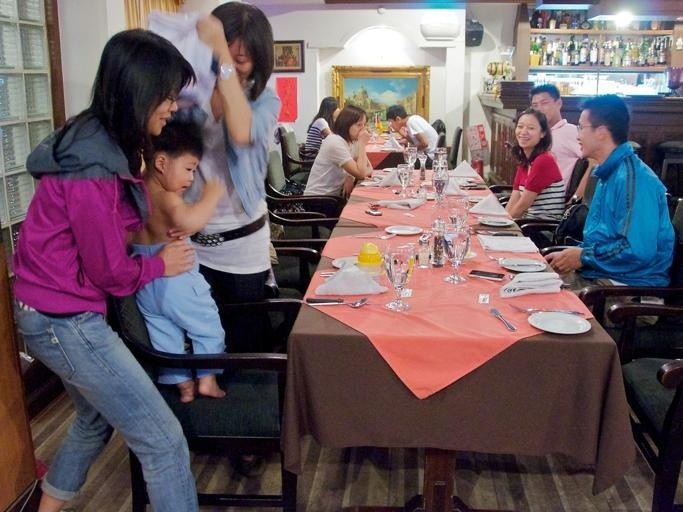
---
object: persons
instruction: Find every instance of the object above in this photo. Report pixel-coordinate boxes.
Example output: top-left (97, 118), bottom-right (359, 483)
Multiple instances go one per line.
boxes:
top-left (173, 2), bottom-right (281, 472)
top-left (545, 96), bottom-right (676, 330)
top-left (529, 85), bottom-right (597, 208)
top-left (507, 108), bottom-right (565, 244)
top-left (384, 105), bottom-right (440, 169)
top-left (304, 97), bottom-right (340, 160)
top-left (303, 106), bottom-right (374, 218)
top-left (133, 118), bottom-right (228, 407)
top-left (12, 30), bottom-right (200, 509)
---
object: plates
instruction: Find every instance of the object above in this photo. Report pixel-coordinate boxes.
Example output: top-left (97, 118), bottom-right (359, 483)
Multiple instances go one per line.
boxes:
top-left (331, 145), bottom-right (593, 211)
top-left (528, 310), bottom-right (593, 335)
top-left (384, 222), bottom-right (424, 236)
top-left (332, 254), bottom-right (393, 273)
top-left (498, 256), bottom-right (548, 273)
top-left (478, 213), bottom-right (516, 227)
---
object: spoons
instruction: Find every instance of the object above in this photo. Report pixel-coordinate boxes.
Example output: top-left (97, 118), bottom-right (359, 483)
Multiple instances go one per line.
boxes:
top-left (307, 296), bottom-right (369, 308)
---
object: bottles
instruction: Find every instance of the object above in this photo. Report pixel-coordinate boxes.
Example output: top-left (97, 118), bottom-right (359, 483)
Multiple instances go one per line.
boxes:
top-left (531, 8), bottom-right (671, 67)
top-left (432, 217), bottom-right (446, 267)
top-left (417, 237), bottom-right (431, 269)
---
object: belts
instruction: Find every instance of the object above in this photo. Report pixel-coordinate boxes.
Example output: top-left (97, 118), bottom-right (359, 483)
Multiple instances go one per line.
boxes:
top-left (17, 300), bottom-right (37, 313)
top-left (189, 212), bottom-right (266, 248)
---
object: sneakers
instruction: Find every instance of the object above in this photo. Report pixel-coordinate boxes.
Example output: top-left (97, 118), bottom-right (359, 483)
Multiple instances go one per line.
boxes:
top-left (232, 438), bottom-right (266, 476)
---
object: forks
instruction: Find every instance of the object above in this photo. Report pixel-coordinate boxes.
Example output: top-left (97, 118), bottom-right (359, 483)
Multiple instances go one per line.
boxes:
top-left (508, 302), bottom-right (585, 316)
top-left (354, 232), bottom-right (396, 241)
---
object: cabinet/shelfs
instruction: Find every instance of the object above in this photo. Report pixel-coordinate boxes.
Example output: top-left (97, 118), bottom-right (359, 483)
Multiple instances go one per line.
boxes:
top-left (530, 29), bottom-right (671, 73)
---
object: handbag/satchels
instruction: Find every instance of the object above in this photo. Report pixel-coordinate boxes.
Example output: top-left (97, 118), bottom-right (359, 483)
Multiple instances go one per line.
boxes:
top-left (554, 196), bottom-right (589, 248)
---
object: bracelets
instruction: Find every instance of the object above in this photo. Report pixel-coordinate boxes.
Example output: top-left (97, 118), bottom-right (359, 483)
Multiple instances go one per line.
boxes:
top-left (572, 193), bottom-right (579, 200)
top-left (217, 63), bottom-right (236, 79)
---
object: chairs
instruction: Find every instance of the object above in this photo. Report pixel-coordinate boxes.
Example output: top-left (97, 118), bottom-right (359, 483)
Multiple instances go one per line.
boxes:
top-left (438, 132), bottom-right (445, 148)
top-left (450, 125), bottom-right (462, 168)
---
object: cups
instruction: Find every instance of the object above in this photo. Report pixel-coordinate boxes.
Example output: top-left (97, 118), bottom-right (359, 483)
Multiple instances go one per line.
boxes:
top-left (499, 45), bottom-right (517, 62)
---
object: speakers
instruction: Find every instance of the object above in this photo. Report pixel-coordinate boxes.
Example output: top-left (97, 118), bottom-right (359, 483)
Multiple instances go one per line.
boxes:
top-left (466, 22), bottom-right (484, 46)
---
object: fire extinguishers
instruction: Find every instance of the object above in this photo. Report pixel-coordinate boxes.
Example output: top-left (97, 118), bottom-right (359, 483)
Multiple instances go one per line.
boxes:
top-left (471, 154), bottom-right (483, 179)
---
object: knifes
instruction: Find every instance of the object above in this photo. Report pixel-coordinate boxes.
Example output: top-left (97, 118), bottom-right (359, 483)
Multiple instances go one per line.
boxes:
top-left (488, 307), bottom-right (519, 333)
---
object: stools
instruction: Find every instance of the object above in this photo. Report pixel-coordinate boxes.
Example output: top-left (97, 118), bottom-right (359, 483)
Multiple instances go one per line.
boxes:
top-left (655, 141), bottom-right (683, 196)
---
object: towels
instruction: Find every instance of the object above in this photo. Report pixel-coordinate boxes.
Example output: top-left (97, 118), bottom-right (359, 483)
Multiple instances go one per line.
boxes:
top-left (379, 170), bottom-right (403, 187)
top-left (476, 235), bottom-right (538, 253)
top-left (378, 198), bottom-right (423, 209)
top-left (469, 193), bottom-right (508, 216)
top-left (449, 160), bottom-right (477, 177)
top-left (315, 261), bottom-right (388, 295)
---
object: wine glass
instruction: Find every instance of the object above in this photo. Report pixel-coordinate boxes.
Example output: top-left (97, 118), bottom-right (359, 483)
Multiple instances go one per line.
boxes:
top-left (447, 198), bottom-right (468, 232)
top-left (442, 223), bottom-right (471, 284)
top-left (384, 243), bottom-right (416, 313)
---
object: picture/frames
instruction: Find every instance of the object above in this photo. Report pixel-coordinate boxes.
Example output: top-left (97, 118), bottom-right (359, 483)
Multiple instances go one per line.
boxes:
top-left (272, 40), bottom-right (304, 72)
top-left (332, 66), bottom-right (429, 123)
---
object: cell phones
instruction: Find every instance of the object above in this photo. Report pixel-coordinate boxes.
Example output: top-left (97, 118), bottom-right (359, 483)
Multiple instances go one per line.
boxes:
top-left (469, 270), bottom-right (505, 281)
